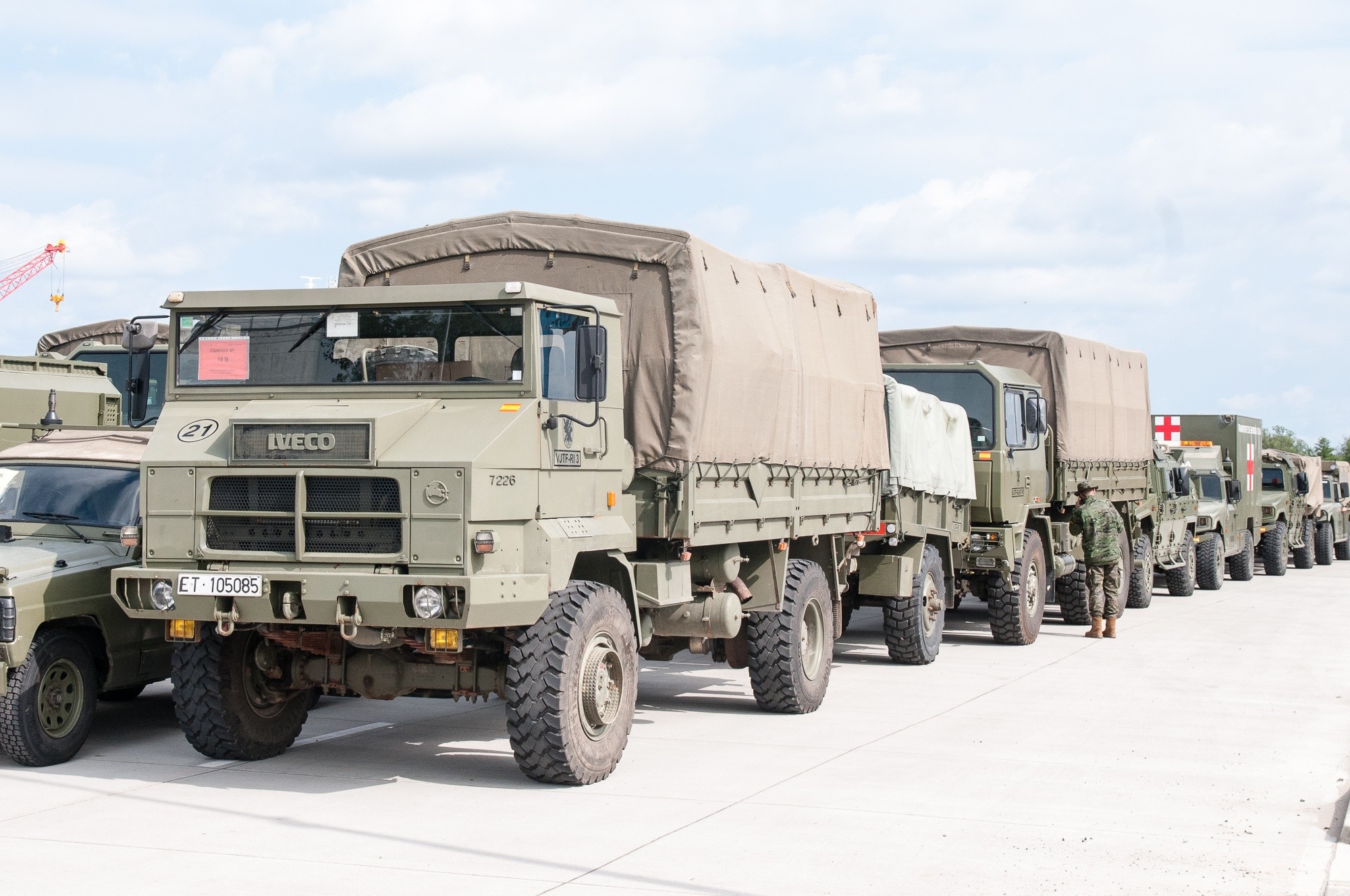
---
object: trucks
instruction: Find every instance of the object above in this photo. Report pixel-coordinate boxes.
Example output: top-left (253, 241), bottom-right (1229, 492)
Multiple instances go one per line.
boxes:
top-left (1150, 412), bottom-right (1266, 584)
top-left (860, 376), bottom-right (975, 661)
top-left (1312, 460), bottom-right (1349, 564)
top-left (103, 211), bottom-right (897, 787)
top-left (34, 317), bottom-right (191, 427)
top-left (0, 423), bottom-right (177, 764)
top-left (866, 320), bottom-right (1153, 636)
top-left (1255, 447), bottom-right (1323, 574)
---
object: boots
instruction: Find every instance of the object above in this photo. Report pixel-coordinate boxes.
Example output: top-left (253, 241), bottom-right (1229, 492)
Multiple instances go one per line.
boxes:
top-left (1086, 616), bottom-right (1103, 638)
top-left (1102, 617), bottom-right (1116, 638)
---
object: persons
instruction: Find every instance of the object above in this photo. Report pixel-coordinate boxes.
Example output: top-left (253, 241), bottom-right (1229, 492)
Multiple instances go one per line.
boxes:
top-left (1069, 482), bottom-right (1123, 639)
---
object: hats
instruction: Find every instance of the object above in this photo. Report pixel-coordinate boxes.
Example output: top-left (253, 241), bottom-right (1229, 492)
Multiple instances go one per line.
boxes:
top-left (1074, 481), bottom-right (1098, 497)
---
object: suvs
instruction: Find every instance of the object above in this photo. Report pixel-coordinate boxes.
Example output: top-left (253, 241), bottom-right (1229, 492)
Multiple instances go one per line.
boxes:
top-left (1151, 441), bottom-right (1203, 595)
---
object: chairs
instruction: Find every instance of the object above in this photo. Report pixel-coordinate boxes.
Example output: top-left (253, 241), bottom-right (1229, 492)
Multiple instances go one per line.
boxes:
top-left (511, 345), bottom-right (566, 395)
